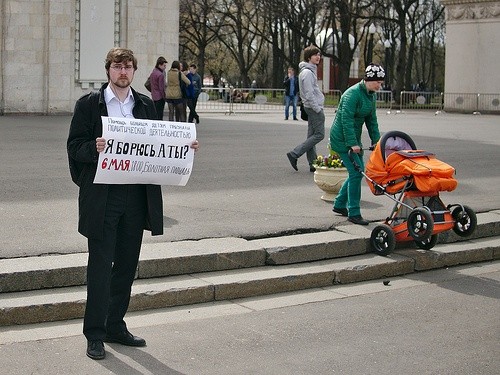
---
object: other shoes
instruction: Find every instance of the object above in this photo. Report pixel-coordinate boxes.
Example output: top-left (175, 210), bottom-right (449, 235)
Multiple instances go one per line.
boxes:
top-left (287, 153), bottom-right (298, 171)
top-left (310, 168), bottom-right (315, 172)
top-left (347, 217), bottom-right (369, 225)
top-left (333, 207), bottom-right (348, 216)
top-left (196, 116), bottom-right (199, 123)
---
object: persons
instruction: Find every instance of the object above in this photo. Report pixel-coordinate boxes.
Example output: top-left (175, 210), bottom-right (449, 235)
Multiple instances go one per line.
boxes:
top-left (67, 48), bottom-right (199, 360)
top-left (286, 47), bottom-right (326, 173)
top-left (166, 61), bottom-right (191, 121)
top-left (227, 85), bottom-right (237, 103)
top-left (251, 80), bottom-right (256, 98)
top-left (283, 67), bottom-right (299, 120)
top-left (186, 64), bottom-right (202, 124)
top-left (180, 62), bottom-right (190, 118)
top-left (150, 57), bottom-right (167, 120)
top-left (329, 64), bottom-right (385, 225)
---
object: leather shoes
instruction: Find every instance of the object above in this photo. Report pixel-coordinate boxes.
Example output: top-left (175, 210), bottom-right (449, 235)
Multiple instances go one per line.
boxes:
top-left (102, 329), bottom-right (146, 347)
top-left (86, 339), bottom-right (106, 360)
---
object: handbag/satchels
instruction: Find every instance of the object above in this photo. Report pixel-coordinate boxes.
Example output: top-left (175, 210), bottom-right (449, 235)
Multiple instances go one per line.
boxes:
top-left (179, 71), bottom-right (187, 94)
top-left (144, 77), bottom-right (152, 92)
top-left (300, 102), bottom-right (309, 121)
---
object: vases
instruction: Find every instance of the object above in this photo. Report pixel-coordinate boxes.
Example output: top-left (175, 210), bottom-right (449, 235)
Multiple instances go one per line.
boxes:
top-left (314, 165), bottom-right (349, 201)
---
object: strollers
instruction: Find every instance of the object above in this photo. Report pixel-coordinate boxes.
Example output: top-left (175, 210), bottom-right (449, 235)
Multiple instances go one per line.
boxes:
top-left (348, 130), bottom-right (478, 257)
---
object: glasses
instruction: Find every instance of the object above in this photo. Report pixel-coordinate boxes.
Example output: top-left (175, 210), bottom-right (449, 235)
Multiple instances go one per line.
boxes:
top-left (109, 65), bottom-right (134, 71)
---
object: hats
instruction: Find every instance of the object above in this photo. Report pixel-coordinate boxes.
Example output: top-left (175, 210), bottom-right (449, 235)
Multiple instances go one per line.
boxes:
top-left (364, 63), bottom-right (386, 81)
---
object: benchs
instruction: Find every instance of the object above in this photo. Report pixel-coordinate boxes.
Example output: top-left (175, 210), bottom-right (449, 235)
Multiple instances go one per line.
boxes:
top-left (234, 92), bottom-right (249, 102)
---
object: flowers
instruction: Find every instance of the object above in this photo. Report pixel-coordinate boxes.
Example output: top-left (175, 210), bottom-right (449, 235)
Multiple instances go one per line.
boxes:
top-left (314, 143), bottom-right (349, 168)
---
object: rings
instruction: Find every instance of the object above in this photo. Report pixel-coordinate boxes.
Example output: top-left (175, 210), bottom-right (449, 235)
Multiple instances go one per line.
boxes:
top-left (97, 145), bottom-right (98, 148)
